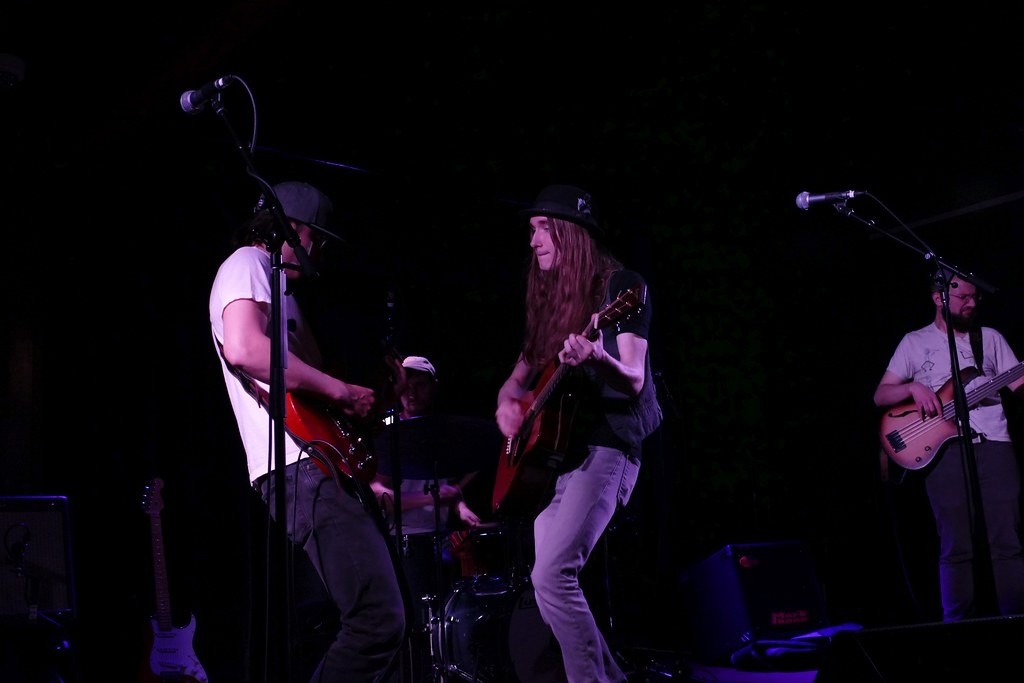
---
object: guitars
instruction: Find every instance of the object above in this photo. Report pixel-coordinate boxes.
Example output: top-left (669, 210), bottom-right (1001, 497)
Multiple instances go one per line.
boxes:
top-left (284, 348), bottom-right (399, 485)
top-left (140, 476), bottom-right (209, 683)
top-left (879, 359), bottom-right (1024, 472)
top-left (490, 286), bottom-right (647, 523)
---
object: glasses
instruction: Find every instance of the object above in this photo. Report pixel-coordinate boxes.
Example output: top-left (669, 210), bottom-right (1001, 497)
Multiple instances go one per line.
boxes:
top-left (949, 291), bottom-right (982, 302)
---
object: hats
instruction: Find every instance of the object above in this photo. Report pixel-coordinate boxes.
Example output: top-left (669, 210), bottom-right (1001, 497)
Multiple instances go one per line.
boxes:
top-left (255, 183), bottom-right (343, 242)
top-left (529, 183), bottom-right (603, 239)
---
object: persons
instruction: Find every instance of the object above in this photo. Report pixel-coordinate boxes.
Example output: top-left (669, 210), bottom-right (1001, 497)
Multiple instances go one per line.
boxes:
top-left (371, 355), bottom-right (482, 531)
top-left (207, 182), bottom-right (409, 683)
top-left (498, 178), bottom-right (663, 683)
top-left (874, 269), bottom-right (1022, 623)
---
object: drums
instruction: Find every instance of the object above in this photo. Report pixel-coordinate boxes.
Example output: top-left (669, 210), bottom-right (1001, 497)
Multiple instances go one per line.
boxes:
top-left (430, 575), bottom-right (565, 683)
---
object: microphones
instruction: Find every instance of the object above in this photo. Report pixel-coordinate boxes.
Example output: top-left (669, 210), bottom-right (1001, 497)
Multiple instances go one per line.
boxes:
top-left (795, 190), bottom-right (868, 211)
top-left (180, 75), bottom-right (232, 114)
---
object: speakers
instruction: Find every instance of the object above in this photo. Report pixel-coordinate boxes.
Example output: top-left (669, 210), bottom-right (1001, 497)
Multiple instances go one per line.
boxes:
top-left (683, 538), bottom-right (823, 658)
